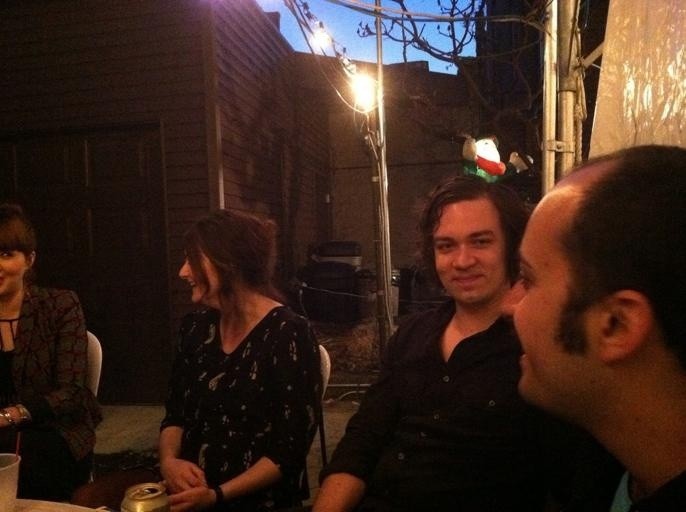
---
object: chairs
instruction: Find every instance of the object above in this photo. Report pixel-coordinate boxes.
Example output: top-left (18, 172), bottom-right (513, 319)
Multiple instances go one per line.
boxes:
top-left (85, 330), bottom-right (103, 398)
top-left (319, 344), bottom-right (331, 401)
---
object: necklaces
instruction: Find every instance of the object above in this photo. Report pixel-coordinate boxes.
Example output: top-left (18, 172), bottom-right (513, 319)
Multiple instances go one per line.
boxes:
top-left (1, 315), bottom-right (20, 346)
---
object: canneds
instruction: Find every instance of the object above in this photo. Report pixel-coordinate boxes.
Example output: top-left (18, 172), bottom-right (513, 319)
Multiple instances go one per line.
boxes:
top-left (122, 482), bottom-right (171, 512)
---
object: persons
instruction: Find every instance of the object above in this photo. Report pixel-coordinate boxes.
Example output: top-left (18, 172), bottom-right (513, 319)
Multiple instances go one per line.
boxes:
top-left (0, 202), bottom-right (105, 504)
top-left (498, 144), bottom-right (685, 511)
top-left (311, 174), bottom-right (628, 511)
top-left (158, 208), bottom-right (322, 510)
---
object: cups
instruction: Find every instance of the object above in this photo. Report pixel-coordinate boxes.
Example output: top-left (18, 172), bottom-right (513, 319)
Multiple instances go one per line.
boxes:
top-left (0, 452), bottom-right (20, 512)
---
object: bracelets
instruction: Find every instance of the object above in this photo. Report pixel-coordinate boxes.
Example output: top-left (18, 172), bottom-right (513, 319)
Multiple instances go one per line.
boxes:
top-left (214, 485), bottom-right (227, 511)
top-left (0, 407), bottom-right (15, 428)
top-left (12, 399), bottom-right (30, 425)
